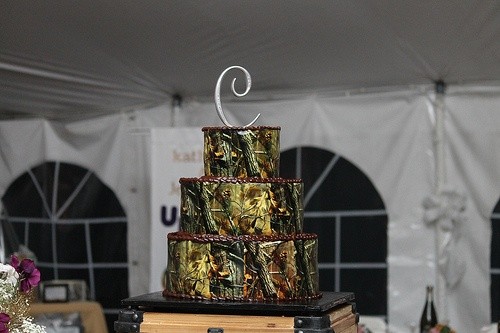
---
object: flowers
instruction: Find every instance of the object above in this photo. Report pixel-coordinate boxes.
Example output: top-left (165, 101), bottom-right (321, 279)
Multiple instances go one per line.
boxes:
top-left (0, 255), bottom-right (48, 333)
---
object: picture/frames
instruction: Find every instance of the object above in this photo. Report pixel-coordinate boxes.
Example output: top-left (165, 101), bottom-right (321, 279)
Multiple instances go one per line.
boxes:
top-left (43, 283), bottom-right (69, 303)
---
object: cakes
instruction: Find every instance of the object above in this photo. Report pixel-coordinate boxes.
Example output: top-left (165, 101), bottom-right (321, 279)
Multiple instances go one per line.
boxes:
top-left (163, 126), bottom-right (323, 303)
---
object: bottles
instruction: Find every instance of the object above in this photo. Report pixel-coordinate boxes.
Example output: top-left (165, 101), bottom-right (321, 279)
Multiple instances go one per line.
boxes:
top-left (419, 285), bottom-right (437, 333)
